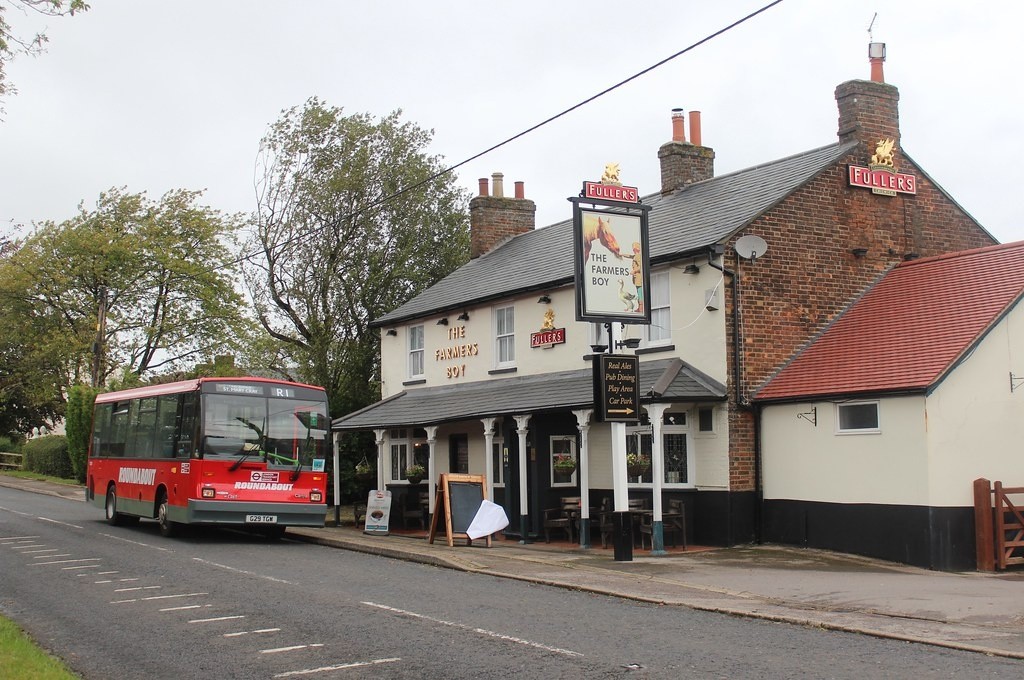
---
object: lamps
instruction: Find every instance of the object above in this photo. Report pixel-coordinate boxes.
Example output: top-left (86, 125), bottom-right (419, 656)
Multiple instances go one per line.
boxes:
top-left (853, 249), bottom-right (868, 256)
top-left (905, 254), bottom-right (919, 261)
top-left (386, 330), bottom-right (396, 336)
top-left (683, 265), bottom-right (700, 274)
top-left (457, 313), bottom-right (469, 320)
top-left (537, 295), bottom-right (551, 304)
top-left (436, 318), bottom-right (448, 325)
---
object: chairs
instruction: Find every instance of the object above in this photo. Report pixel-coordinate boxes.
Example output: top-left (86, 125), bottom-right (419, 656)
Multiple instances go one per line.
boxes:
top-left (542, 496), bottom-right (686, 552)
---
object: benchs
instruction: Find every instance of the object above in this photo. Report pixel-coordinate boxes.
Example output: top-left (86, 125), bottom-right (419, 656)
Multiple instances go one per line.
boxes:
top-left (354, 483), bottom-right (436, 530)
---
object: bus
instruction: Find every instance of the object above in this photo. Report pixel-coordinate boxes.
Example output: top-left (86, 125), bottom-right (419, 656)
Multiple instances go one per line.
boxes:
top-left (83, 376), bottom-right (336, 542)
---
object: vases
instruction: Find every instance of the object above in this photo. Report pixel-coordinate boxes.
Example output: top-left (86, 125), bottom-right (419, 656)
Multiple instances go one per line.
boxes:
top-left (406, 474), bottom-right (424, 483)
top-left (356, 473), bottom-right (371, 479)
top-left (627, 465), bottom-right (649, 477)
top-left (667, 471), bottom-right (679, 483)
top-left (554, 467), bottom-right (575, 476)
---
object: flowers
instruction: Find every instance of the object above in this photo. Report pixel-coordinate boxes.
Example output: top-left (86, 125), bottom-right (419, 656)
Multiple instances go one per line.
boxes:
top-left (626, 453), bottom-right (650, 466)
top-left (668, 449), bottom-right (681, 472)
top-left (355, 465), bottom-right (372, 474)
top-left (554, 453), bottom-right (576, 468)
top-left (405, 464), bottom-right (425, 476)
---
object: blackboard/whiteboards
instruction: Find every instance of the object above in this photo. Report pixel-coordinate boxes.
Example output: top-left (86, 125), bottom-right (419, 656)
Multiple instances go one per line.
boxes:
top-left (430, 474), bottom-right (491, 538)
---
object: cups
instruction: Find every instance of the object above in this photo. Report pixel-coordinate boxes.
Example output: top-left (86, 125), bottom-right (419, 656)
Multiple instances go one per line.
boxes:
top-left (370, 510), bottom-right (384, 523)
